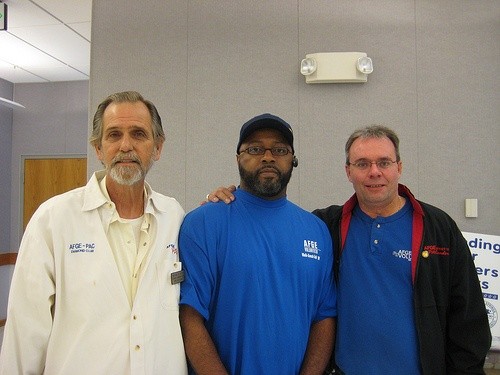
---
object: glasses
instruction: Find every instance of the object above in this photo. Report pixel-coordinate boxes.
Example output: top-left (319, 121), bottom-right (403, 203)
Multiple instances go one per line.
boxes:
top-left (240, 146), bottom-right (293, 157)
top-left (348, 160), bottom-right (397, 169)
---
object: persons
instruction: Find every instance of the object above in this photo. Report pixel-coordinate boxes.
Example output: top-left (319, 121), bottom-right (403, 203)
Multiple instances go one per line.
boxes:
top-left (178, 114), bottom-right (338, 375)
top-left (198, 125), bottom-right (493, 375)
top-left (0, 90), bottom-right (189, 375)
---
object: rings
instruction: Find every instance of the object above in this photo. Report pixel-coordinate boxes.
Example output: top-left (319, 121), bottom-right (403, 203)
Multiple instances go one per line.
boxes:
top-left (206, 193), bottom-right (211, 201)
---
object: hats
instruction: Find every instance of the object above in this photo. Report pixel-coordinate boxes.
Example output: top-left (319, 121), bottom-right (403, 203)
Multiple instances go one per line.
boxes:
top-left (236, 114), bottom-right (294, 155)
